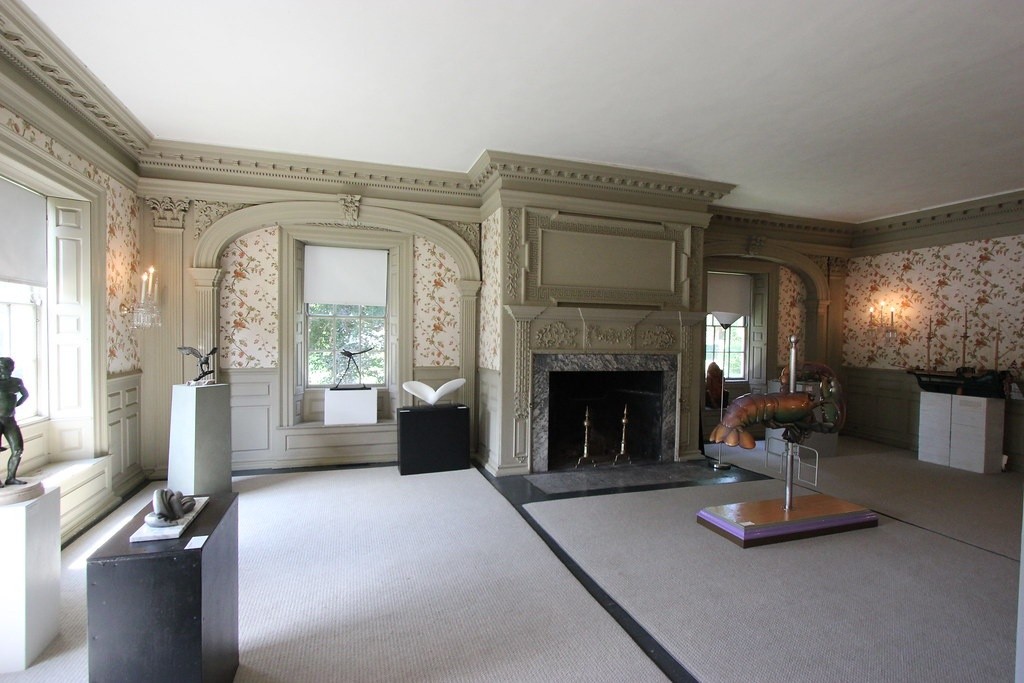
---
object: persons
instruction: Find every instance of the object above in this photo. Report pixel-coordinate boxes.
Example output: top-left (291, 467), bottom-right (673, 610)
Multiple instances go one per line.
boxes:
top-left (0, 357), bottom-right (28, 487)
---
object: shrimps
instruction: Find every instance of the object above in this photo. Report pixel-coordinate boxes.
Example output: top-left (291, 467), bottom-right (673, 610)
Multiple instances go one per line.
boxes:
top-left (709, 360), bottom-right (846, 450)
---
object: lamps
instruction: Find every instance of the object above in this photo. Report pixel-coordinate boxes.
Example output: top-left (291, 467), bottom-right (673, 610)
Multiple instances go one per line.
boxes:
top-left (132, 265), bottom-right (161, 327)
top-left (870, 309), bottom-right (898, 339)
top-left (709, 311), bottom-right (741, 469)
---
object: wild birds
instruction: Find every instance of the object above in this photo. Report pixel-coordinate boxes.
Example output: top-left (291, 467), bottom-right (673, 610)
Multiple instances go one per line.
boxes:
top-left (177, 346), bottom-right (218, 374)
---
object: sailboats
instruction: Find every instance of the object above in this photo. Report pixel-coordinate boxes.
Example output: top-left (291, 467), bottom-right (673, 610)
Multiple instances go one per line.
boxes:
top-left (905, 309), bottom-right (1009, 398)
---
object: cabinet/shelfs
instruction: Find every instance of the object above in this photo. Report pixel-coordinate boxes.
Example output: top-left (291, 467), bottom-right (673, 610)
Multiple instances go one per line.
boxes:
top-left (918, 391), bottom-right (1006, 474)
